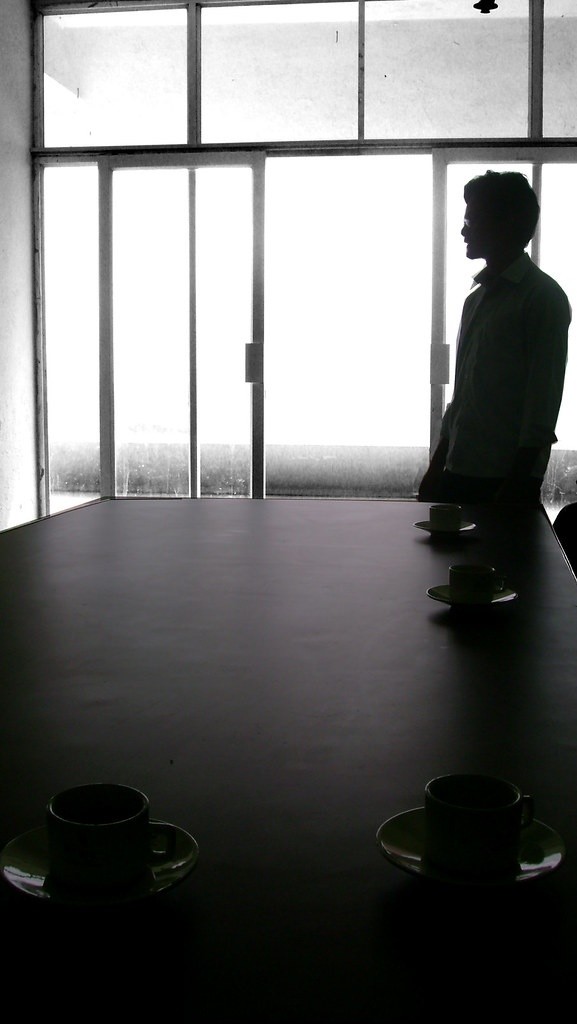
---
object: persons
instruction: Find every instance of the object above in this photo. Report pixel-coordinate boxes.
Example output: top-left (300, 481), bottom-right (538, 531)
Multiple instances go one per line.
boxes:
top-left (417, 170), bottom-right (571, 500)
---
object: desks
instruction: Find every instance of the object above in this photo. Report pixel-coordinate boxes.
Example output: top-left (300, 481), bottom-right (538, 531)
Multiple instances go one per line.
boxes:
top-left (0, 496), bottom-right (577, 1024)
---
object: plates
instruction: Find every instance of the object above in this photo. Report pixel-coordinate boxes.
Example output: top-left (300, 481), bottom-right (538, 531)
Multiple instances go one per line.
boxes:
top-left (428, 585), bottom-right (519, 608)
top-left (0, 818), bottom-right (200, 903)
top-left (415, 522), bottom-right (476, 534)
top-left (376, 806), bottom-right (566, 886)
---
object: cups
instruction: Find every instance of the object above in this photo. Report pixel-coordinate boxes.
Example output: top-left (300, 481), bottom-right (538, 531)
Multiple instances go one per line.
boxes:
top-left (449, 563), bottom-right (505, 604)
top-left (423, 772), bottom-right (536, 877)
top-left (430, 505), bottom-right (463, 530)
top-left (46, 782), bottom-right (175, 898)
